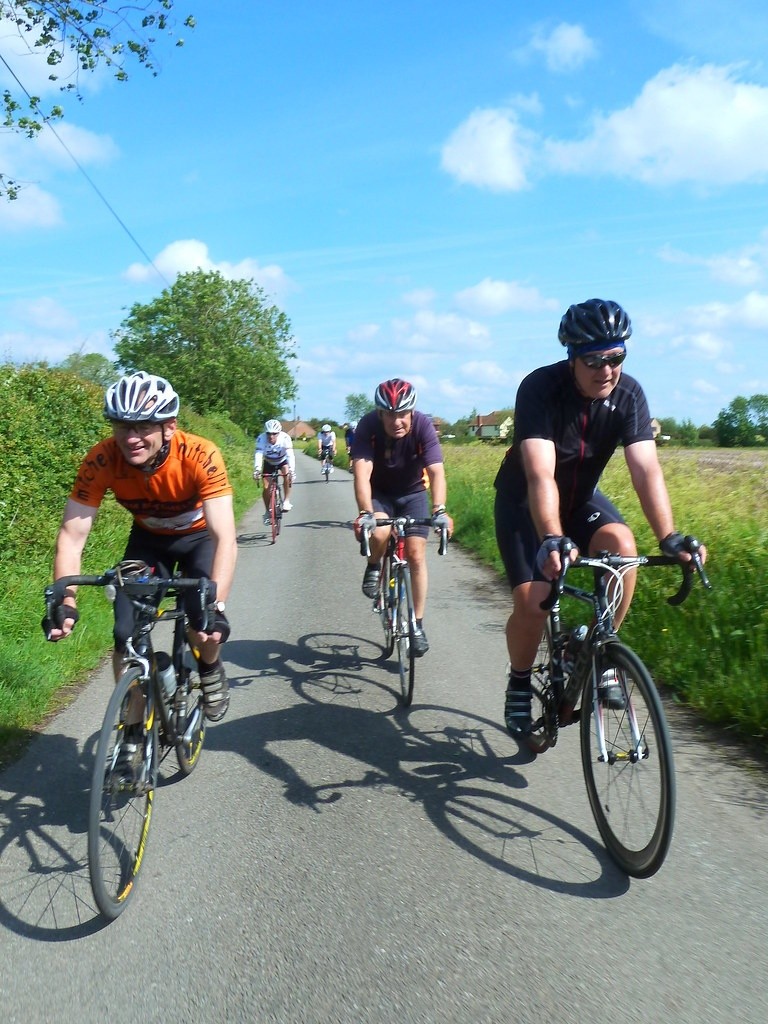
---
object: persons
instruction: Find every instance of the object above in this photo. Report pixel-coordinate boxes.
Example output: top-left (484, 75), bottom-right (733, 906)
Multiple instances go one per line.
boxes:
top-left (491, 300), bottom-right (706, 744)
top-left (317, 423), bottom-right (337, 475)
top-left (252, 419), bottom-right (295, 526)
top-left (350, 379), bottom-right (455, 653)
top-left (345, 421), bottom-right (358, 473)
top-left (41, 370), bottom-right (239, 786)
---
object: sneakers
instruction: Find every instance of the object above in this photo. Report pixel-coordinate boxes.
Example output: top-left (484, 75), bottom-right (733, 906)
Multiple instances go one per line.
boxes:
top-left (198, 661), bottom-right (229, 722)
top-left (112, 742), bottom-right (141, 784)
top-left (410, 627), bottom-right (429, 656)
top-left (263, 512), bottom-right (271, 526)
top-left (505, 684), bottom-right (532, 736)
top-left (282, 501), bottom-right (292, 512)
top-left (596, 668), bottom-right (626, 710)
top-left (361, 563), bottom-right (381, 599)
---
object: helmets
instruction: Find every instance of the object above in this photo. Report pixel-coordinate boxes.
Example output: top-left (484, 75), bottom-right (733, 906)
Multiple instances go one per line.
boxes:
top-left (265, 419), bottom-right (282, 433)
top-left (375, 378), bottom-right (417, 412)
top-left (557, 298), bottom-right (632, 347)
top-left (104, 370), bottom-right (179, 423)
top-left (349, 422), bottom-right (357, 430)
top-left (322, 424), bottom-right (332, 432)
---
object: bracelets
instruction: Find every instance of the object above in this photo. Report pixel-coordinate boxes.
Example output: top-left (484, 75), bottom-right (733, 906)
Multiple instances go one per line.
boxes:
top-left (433, 504), bottom-right (446, 509)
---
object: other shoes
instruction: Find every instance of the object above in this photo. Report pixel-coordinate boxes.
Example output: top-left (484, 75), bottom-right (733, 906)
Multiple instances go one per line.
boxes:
top-left (330, 465), bottom-right (334, 473)
top-left (321, 467), bottom-right (326, 474)
top-left (349, 466), bottom-right (354, 473)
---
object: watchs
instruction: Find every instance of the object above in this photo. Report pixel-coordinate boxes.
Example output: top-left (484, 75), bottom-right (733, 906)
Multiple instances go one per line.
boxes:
top-left (214, 601), bottom-right (226, 613)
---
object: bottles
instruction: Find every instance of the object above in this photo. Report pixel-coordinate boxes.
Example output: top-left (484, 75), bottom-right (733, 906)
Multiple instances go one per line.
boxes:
top-left (560, 625), bottom-right (589, 674)
top-left (151, 651), bottom-right (177, 699)
top-left (388, 578), bottom-right (404, 608)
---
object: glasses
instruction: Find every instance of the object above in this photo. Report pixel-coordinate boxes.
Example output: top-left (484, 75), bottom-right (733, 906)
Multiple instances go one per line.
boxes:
top-left (578, 349), bottom-right (626, 368)
top-left (110, 420), bottom-right (158, 435)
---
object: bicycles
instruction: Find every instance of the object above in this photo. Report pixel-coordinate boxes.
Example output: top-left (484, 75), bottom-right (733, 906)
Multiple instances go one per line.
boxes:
top-left (358, 506), bottom-right (452, 707)
top-left (517, 533), bottom-right (716, 881)
top-left (41, 557), bottom-right (216, 921)
top-left (346, 446), bottom-right (356, 475)
top-left (255, 464), bottom-right (291, 544)
top-left (318, 449), bottom-right (336, 481)
